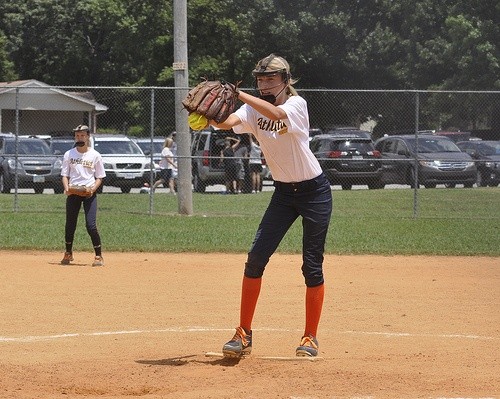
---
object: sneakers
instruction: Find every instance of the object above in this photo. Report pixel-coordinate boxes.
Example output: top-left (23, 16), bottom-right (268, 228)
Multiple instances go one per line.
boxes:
top-left (61, 251), bottom-right (72, 264)
top-left (222, 329), bottom-right (252, 358)
top-left (295, 334), bottom-right (319, 357)
top-left (93, 256), bottom-right (103, 266)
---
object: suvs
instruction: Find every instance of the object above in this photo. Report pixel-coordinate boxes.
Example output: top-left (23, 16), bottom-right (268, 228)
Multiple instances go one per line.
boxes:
top-left (372, 134), bottom-right (479, 189)
top-left (309, 133), bottom-right (386, 191)
top-left (189, 128), bottom-right (270, 194)
top-left (41, 133), bottom-right (180, 195)
top-left (0, 132), bottom-right (64, 193)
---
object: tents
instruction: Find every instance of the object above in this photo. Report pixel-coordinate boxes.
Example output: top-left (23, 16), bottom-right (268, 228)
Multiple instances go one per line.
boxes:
top-left (0, 80), bottom-right (109, 134)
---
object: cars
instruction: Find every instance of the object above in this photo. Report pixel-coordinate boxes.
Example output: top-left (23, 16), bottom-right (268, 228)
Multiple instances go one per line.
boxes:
top-left (309, 126), bottom-right (484, 155)
top-left (455, 140), bottom-right (500, 188)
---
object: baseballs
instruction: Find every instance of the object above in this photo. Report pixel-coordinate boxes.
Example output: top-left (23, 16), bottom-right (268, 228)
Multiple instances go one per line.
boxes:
top-left (188, 112), bottom-right (210, 131)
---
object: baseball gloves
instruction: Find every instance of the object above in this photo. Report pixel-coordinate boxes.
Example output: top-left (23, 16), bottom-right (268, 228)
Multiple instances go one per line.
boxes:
top-left (182, 79), bottom-right (240, 124)
top-left (68, 185), bottom-right (92, 198)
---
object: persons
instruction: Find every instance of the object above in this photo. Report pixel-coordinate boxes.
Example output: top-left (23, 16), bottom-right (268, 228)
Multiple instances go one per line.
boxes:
top-left (150, 138), bottom-right (178, 196)
top-left (248, 133), bottom-right (263, 193)
top-left (171, 132), bottom-right (177, 144)
top-left (181, 52), bottom-right (332, 360)
top-left (231, 136), bottom-right (248, 194)
top-left (61, 124), bottom-right (106, 266)
top-left (219, 136), bottom-right (240, 194)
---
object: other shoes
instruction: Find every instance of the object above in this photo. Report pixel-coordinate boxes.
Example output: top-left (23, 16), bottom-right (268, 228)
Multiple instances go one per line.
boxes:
top-left (149, 188), bottom-right (155, 195)
top-left (170, 191), bottom-right (176, 196)
